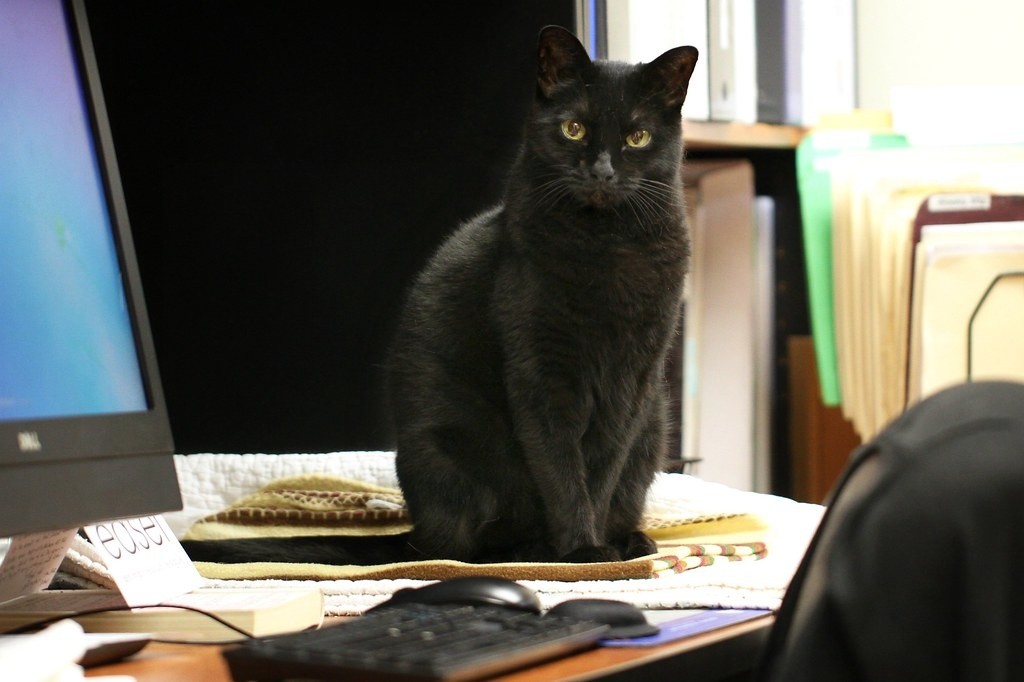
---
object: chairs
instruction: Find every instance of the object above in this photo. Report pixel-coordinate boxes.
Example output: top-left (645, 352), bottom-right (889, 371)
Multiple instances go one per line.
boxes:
top-left (764, 379), bottom-right (1024, 682)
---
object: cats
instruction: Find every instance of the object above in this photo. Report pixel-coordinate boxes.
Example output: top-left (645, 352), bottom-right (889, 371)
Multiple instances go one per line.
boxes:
top-left (178, 24), bottom-right (699, 562)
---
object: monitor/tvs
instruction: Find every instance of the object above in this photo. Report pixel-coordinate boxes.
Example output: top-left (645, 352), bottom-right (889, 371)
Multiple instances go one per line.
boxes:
top-left (0, 0), bottom-right (183, 538)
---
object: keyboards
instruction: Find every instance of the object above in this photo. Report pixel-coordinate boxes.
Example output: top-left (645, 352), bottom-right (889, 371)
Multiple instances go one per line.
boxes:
top-left (223, 600), bottom-right (609, 682)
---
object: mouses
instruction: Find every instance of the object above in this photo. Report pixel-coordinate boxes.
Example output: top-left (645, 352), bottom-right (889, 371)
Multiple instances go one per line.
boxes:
top-left (366, 576), bottom-right (542, 614)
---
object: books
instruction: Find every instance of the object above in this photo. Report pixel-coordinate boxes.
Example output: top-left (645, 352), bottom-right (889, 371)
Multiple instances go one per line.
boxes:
top-left (0, 514), bottom-right (323, 640)
top-left (828, 147), bottom-right (1024, 443)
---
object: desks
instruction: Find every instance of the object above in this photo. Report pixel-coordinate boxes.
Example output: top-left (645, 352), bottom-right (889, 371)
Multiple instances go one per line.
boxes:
top-left (85, 610), bottom-right (777, 682)
